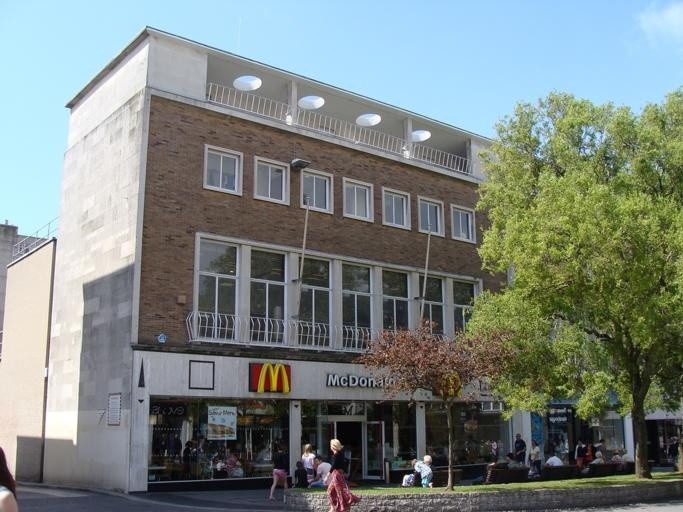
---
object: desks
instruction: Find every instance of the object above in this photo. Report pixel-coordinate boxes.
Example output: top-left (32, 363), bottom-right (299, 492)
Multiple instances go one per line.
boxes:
top-left (148, 466), bottom-right (167, 482)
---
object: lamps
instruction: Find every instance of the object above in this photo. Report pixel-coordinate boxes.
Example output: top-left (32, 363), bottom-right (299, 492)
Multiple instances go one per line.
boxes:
top-left (289, 157), bottom-right (312, 170)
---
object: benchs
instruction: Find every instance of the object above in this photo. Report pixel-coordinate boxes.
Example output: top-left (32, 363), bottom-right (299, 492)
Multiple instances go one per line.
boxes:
top-left (406, 461), bottom-right (653, 486)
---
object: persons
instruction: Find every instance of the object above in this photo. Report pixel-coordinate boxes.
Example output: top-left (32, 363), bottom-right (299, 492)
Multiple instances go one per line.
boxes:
top-left (268, 442), bottom-right (290, 501)
top-left (323, 437), bottom-right (362, 512)
top-left (291, 443), bottom-right (333, 488)
top-left (666, 436), bottom-right (682, 473)
top-left (574, 439), bottom-right (635, 474)
top-left (0, 447), bottom-right (20, 512)
top-left (483, 433), bottom-right (563, 480)
top-left (400, 454), bottom-right (442, 488)
top-left (171, 435), bottom-right (245, 481)
